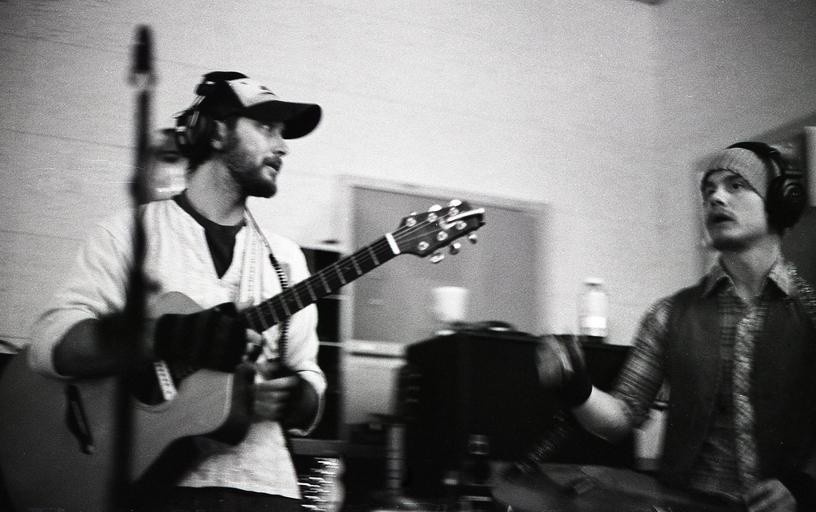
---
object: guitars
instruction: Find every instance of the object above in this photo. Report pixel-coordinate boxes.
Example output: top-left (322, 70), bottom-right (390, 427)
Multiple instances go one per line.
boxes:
top-left (1, 197), bottom-right (487, 510)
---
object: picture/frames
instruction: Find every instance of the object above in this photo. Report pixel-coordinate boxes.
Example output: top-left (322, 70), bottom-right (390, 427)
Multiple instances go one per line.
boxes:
top-left (335, 174), bottom-right (548, 359)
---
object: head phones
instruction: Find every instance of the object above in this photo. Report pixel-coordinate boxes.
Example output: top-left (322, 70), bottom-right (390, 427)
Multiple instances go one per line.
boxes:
top-left (174, 71), bottom-right (247, 155)
top-left (727, 142), bottom-right (806, 230)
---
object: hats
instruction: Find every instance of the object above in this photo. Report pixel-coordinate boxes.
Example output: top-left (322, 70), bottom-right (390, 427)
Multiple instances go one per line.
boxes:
top-left (700, 147), bottom-right (780, 200)
top-left (180, 71), bottom-right (319, 139)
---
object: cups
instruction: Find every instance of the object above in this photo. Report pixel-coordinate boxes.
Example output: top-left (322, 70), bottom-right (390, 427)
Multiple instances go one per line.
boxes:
top-left (431, 284), bottom-right (468, 336)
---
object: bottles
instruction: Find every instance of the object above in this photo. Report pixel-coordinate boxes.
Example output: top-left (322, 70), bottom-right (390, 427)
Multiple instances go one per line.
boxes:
top-left (574, 277), bottom-right (609, 339)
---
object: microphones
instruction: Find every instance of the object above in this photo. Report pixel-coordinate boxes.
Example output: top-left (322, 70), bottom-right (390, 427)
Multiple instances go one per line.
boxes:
top-left (126, 23), bottom-right (159, 90)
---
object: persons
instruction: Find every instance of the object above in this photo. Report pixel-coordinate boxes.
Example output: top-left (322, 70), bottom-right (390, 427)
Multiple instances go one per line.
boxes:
top-left (26, 71), bottom-right (329, 511)
top-left (539, 141), bottom-right (816, 512)
